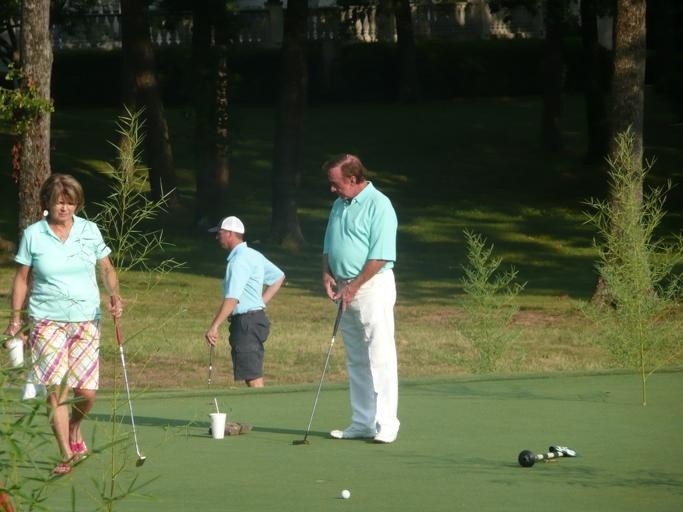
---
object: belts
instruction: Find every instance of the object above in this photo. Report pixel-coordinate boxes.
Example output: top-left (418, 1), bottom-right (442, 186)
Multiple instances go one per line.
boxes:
top-left (334, 277), bottom-right (356, 285)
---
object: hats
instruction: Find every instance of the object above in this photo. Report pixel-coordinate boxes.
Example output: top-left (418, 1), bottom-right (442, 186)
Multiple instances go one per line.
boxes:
top-left (207, 215), bottom-right (244, 235)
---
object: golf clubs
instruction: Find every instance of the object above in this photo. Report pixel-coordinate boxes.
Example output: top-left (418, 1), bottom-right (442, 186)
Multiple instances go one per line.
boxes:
top-left (111, 295), bottom-right (147, 466)
top-left (208, 342), bottom-right (215, 438)
top-left (293, 299), bottom-right (343, 445)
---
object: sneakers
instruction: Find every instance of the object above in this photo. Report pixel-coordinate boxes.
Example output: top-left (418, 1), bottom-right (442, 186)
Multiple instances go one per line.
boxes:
top-left (69, 438), bottom-right (88, 453)
top-left (52, 461), bottom-right (70, 474)
top-left (373, 418), bottom-right (400, 444)
top-left (330, 420), bottom-right (376, 439)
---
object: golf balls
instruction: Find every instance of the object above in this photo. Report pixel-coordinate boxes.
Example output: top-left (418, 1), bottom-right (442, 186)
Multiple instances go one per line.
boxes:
top-left (342, 489), bottom-right (350, 499)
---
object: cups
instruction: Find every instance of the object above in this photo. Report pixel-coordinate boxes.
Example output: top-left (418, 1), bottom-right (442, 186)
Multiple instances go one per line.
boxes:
top-left (207, 413), bottom-right (226, 439)
top-left (2, 338), bottom-right (24, 369)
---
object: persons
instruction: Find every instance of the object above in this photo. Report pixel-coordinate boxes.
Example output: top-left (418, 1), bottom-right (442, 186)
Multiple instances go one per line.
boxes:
top-left (204, 215), bottom-right (284, 388)
top-left (2, 171), bottom-right (123, 475)
top-left (321, 155), bottom-right (399, 442)
